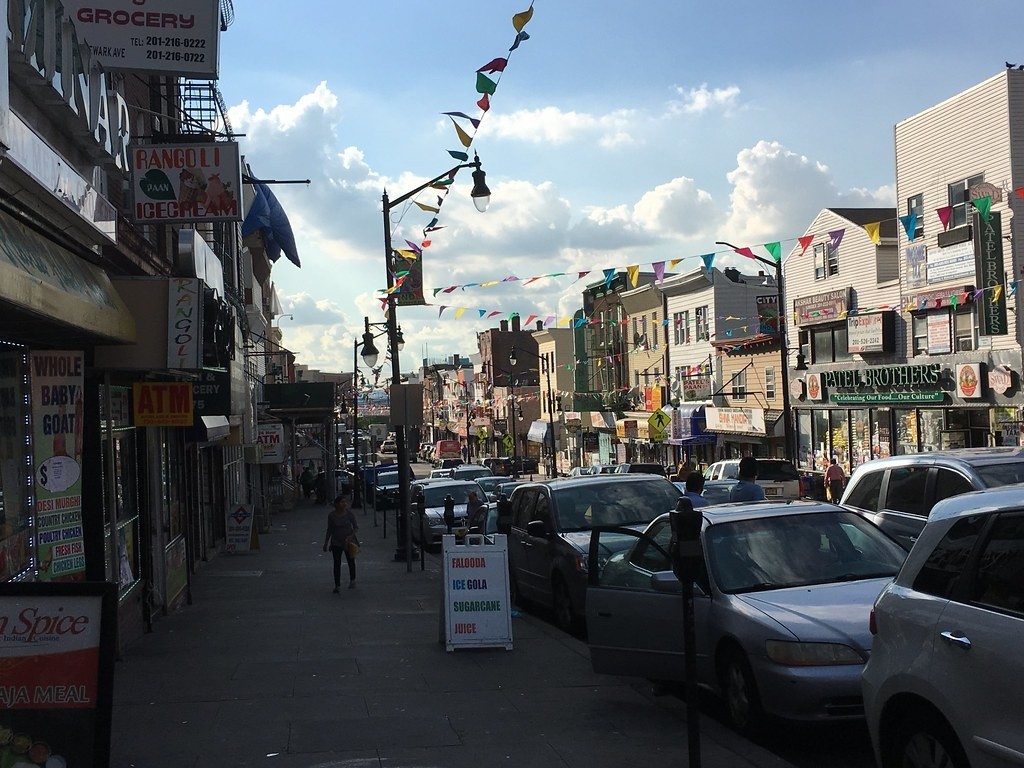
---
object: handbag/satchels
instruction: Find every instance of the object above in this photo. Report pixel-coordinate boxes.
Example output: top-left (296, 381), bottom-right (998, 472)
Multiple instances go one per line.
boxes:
top-left (349, 542), bottom-right (361, 558)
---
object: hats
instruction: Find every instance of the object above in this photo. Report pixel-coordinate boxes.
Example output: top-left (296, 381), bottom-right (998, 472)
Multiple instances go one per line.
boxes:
top-left (739, 457), bottom-right (760, 476)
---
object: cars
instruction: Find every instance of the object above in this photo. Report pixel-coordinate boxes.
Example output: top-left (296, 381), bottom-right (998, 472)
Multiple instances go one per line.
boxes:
top-left (312, 470), bottom-right (356, 490)
top-left (584, 499), bottom-right (909, 736)
top-left (337, 437), bottom-right (397, 473)
top-left (672, 481), bottom-right (768, 508)
top-left (474, 475), bottom-right (529, 503)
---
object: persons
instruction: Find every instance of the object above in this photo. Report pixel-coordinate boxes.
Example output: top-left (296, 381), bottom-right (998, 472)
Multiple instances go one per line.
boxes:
top-left (466, 491), bottom-right (485, 534)
top-left (462, 444), bottom-right (467, 463)
top-left (682, 472), bottom-right (709, 508)
top-left (511, 459), bottom-right (519, 479)
top-left (323, 496), bottom-right (360, 594)
top-left (729, 458), bottom-right (765, 502)
top-left (283, 434), bottom-right (323, 500)
top-left (824, 458), bottom-right (847, 505)
top-left (543, 455), bottom-right (550, 479)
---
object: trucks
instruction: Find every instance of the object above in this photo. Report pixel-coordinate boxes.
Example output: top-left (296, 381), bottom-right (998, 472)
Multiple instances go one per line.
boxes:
top-left (367, 424), bottom-right (386, 445)
top-left (435, 440), bottom-right (460, 463)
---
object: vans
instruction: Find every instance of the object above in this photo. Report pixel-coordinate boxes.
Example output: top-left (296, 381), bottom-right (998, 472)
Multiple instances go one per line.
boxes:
top-left (569, 463), bottom-right (665, 478)
top-left (502, 473), bottom-right (691, 640)
top-left (702, 457), bottom-right (803, 502)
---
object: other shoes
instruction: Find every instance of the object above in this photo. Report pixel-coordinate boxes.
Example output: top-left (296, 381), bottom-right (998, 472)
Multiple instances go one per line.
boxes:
top-left (349, 579), bottom-right (356, 588)
top-left (333, 586), bottom-right (341, 592)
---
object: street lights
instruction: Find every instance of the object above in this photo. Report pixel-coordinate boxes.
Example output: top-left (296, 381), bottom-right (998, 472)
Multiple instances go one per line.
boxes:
top-left (364, 148), bottom-right (490, 562)
top-left (509, 345), bottom-right (563, 478)
top-left (442, 360), bottom-right (524, 479)
top-left (352, 325), bottom-right (405, 508)
top-left (781, 342), bottom-right (809, 458)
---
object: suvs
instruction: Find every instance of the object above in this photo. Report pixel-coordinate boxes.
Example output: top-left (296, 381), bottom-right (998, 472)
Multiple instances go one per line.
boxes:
top-left (837, 448), bottom-right (1024, 555)
top-left (410, 478), bottom-right (491, 552)
top-left (450, 456), bottom-right (512, 480)
top-left (861, 481), bottom-right (1024, 768)
top-left (370, 471), bottom-right (398, 507)
